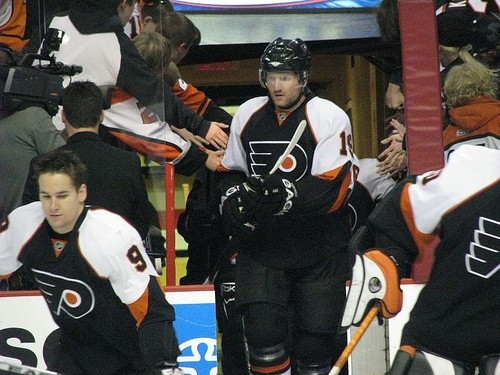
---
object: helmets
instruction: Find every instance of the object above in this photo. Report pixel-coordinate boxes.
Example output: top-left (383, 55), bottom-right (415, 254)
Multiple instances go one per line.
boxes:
top-left (259, 37), bottom-right (310, 87)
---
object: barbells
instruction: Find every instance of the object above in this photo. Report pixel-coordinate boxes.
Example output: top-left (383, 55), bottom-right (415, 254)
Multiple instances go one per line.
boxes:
top-left (208, 119), bottom-right (306, 282)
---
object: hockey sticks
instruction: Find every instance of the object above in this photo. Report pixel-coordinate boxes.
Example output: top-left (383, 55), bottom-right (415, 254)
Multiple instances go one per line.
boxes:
top-left (326, 300), bottom-right (382, 375)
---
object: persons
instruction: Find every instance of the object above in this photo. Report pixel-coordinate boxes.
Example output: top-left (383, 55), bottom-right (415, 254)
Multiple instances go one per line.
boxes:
top-left (0, 0), bottom-right (233, 290)
top-left (212, 37), bottom-right (360, 375)
top-left (375, 0), bottom-right (500, 181)
top-left (346, 143), bottom-right (500, 375)
top-left (0, 149), bottom-right (182, 375)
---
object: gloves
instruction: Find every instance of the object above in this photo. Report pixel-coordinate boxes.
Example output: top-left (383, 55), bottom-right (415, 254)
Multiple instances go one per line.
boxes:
top-left (222, 191), bottom-right (260, 242)
top-left (238, 174), bottom-right (301, 217)
